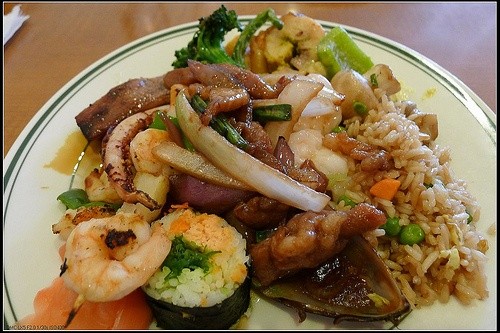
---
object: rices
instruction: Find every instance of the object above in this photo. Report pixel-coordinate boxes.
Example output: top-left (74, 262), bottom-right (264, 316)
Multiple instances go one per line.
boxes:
top-left (337, 96), bottom-right (489, 309)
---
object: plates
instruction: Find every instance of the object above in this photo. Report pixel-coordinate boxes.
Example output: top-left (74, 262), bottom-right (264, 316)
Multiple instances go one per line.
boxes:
top-left (0, 15), bottom-right (497, 330)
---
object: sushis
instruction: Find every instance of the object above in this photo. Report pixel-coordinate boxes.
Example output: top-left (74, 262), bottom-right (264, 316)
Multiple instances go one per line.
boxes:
top-left (137, 206), bottom-right (250, 329)
